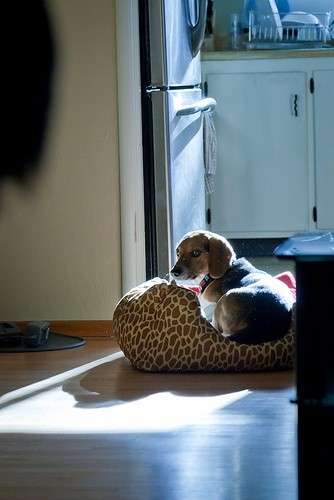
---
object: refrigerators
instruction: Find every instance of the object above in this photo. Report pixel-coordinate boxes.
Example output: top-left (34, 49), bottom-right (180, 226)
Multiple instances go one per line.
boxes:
top-left (138, 0), bottom-right (217, 282)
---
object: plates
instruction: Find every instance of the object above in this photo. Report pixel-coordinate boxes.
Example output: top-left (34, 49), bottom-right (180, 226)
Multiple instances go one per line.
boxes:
top-left (254, 0), bottom-right (284, 41)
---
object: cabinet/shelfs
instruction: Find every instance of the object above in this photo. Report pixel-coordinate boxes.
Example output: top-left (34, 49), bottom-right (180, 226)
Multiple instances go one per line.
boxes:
top-left (274, 232), bottom-right (334, 500)
top-left (201, 57), bottom-right (334, 239)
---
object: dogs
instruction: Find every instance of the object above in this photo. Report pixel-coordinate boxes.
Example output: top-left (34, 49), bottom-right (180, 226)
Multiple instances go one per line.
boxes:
top-left (168, 226), bottom-right (296, 344)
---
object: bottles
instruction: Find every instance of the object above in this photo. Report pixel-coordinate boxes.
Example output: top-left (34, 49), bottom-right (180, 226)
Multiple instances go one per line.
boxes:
top-left (229, 14), bottom-right (243, 51)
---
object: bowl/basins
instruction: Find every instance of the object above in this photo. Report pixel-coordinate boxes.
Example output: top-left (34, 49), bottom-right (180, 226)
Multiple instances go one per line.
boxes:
top-left (280, 12), bottom-right (320, 24)
top-left (297, 24), bottom-right (330, 40)
